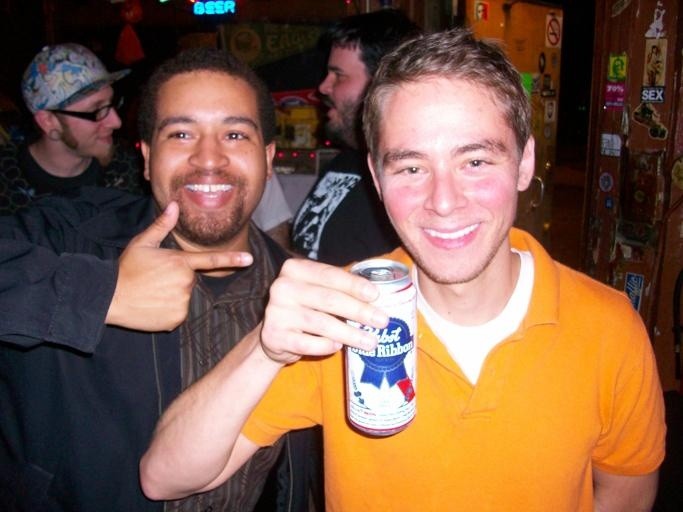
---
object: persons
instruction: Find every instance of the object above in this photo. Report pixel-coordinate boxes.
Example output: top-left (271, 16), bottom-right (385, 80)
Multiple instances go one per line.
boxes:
top-left (2, 43), bottom-right (150, 214)
top-left (140, 30), bottom-right (666, 511)
top-left (247, 170), bottom-right (294, 253)
top-left (3, 46), bottom-right (326, 512)
top-left (295, 12), bottom-right (418, 264)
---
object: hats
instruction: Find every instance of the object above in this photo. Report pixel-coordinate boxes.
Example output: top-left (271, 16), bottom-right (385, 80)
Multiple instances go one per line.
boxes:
top-left (22, 42), bottom-right (131, 114)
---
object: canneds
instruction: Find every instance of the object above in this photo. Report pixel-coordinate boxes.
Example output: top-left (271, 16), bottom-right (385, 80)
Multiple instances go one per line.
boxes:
top-left (344, 258), bottom-right (418, 437)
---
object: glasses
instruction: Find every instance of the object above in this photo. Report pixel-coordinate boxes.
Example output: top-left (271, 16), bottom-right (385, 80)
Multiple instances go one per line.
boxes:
top-left (46, 95), bottom-right (124, 121)
